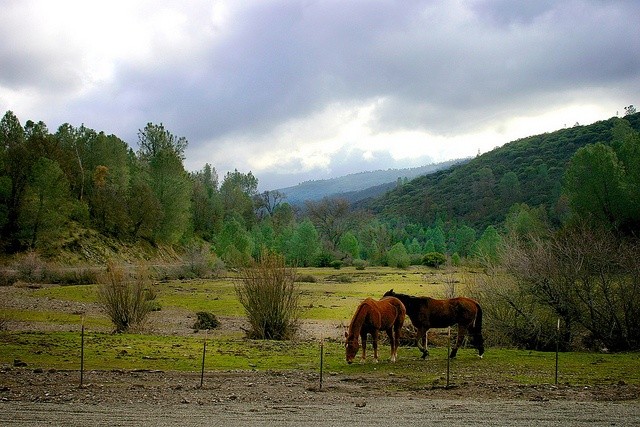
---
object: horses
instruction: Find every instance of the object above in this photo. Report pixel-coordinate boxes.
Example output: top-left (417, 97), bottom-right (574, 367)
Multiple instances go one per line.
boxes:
top-left (345, 296), bottom-right (406, 364)
top-left (382, 289), bottom-right (484, 360)
